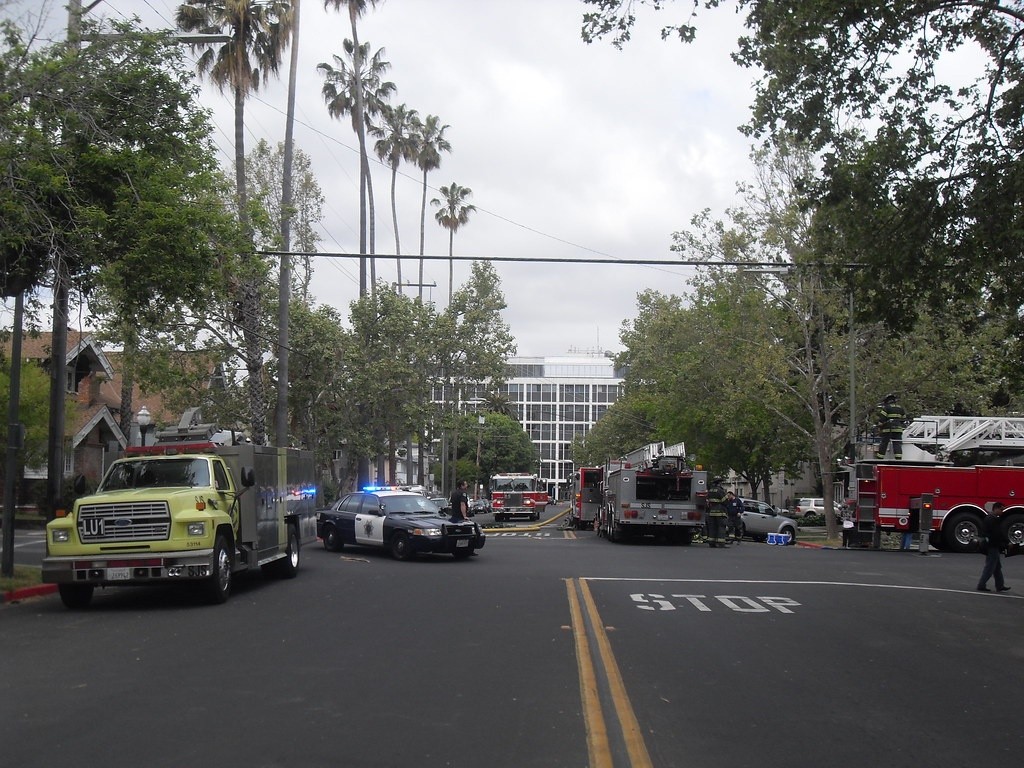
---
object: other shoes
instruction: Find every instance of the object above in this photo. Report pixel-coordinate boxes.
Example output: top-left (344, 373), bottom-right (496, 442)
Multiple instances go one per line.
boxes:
top-left (996, 587), bottom-right (1011, 592)
top-left (737, 540), bottom-right (741, 545)
top-left (471, 553), bottom-right (479, 557)
top-left (709, 544), bottom-right (725, 548)
top-left (977, 586), bottom-right (991, 592)
top-left (728, 540), bottom-right (733, 544)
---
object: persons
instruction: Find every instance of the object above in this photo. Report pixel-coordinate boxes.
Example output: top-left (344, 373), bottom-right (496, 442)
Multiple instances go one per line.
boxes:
top-left (772, 505), bottom-right (777, 512)
top-left (451, 479), bottom-right (479, 556)
top-left (852, 503), bottom-right (857, 518)
top-left (785, 496), bottom-right (791, 510)
top-left (692, 476), bottom-right (745, 548)
top-left (977, 502), bottom-right (1012, 592)
top-left (900, 533), bottom-right (913, 550)
top-left (875, 394), bottom-right (906, 460)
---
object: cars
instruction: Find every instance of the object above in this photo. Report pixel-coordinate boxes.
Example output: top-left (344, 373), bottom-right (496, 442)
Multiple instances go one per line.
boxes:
top-left (548, 495), bottom-right (557, 505)
top-left (727, 498), bottom-right (797, 546)
top-left (313, 486), bottom-right (487, 562)
top-left (405, 483), bottom-right (491, 517)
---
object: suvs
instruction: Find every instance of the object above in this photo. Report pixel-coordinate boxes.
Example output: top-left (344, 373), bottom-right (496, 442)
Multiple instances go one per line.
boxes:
top-left (795, 497), bottom-right (842, 520)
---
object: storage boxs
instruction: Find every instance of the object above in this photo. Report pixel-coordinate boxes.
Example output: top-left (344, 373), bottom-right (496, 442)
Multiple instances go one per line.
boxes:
top-left (766, 533), bottom-right (790, 546)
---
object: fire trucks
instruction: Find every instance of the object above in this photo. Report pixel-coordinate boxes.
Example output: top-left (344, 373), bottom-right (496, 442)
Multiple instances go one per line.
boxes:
top-left (834, 412), bottom-right (1024, 558)
top-left (490, 471), bottom-right (549, 521)
top-left (41, 407), bottom-right (320, 611)
top-left (565, 440), bottom-right (709, 544)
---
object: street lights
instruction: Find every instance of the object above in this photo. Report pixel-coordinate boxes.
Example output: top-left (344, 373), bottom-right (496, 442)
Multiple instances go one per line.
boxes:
top-left (136, 405), bottom-right (152, 447)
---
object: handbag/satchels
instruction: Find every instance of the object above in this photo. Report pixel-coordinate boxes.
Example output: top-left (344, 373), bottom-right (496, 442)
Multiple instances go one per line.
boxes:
top-left (974, 541), bottom-right (989, 556)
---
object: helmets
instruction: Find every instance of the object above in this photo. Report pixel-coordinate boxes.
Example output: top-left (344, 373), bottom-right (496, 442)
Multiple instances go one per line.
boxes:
top-left (710, 476), bottom-right (724, 483)
